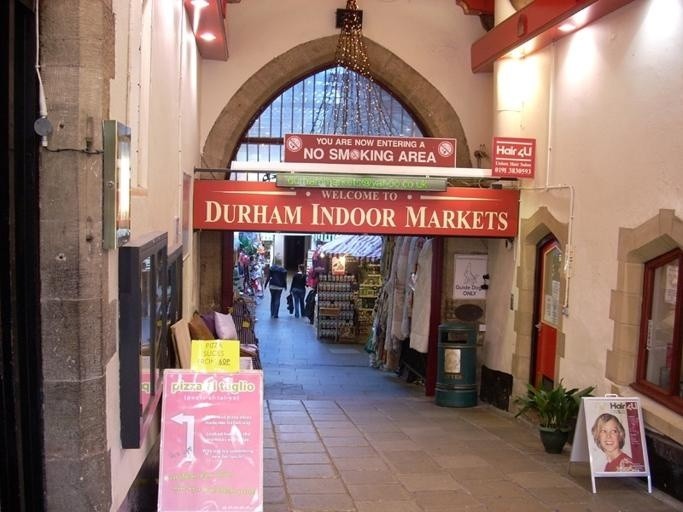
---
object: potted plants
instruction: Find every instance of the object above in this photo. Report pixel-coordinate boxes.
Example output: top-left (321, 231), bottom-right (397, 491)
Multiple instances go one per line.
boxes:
top-left (508, 372), bottom-right (597, 455)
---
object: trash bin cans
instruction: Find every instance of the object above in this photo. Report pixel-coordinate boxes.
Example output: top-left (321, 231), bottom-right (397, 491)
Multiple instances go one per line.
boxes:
top-left (434, 323), bottom-right (478, 407)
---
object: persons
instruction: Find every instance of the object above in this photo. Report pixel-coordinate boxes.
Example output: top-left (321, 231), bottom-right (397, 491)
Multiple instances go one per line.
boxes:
top-left (263, 259), bottom-right (287, 319)
top-left (590, 412), bottom-right (643, 472)
top-left (289, 264), bottom-right (306, 317)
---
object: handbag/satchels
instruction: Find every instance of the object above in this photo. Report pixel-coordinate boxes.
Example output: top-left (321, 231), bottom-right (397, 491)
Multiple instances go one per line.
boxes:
top-left (287, 292), bottom-right (294, 314)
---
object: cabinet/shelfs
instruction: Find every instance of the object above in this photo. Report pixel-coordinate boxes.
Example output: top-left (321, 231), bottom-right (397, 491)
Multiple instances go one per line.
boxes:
top-left (313, 255), bottom-right (383, 344)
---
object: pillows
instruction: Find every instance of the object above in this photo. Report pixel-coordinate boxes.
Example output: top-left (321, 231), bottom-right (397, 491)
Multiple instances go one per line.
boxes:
top-left (212, 310), bottom-right (237, 340)
top-left (199, 310), bottom-right (216, 335)
top-left (186, 316), bottom-right (214, 342)
